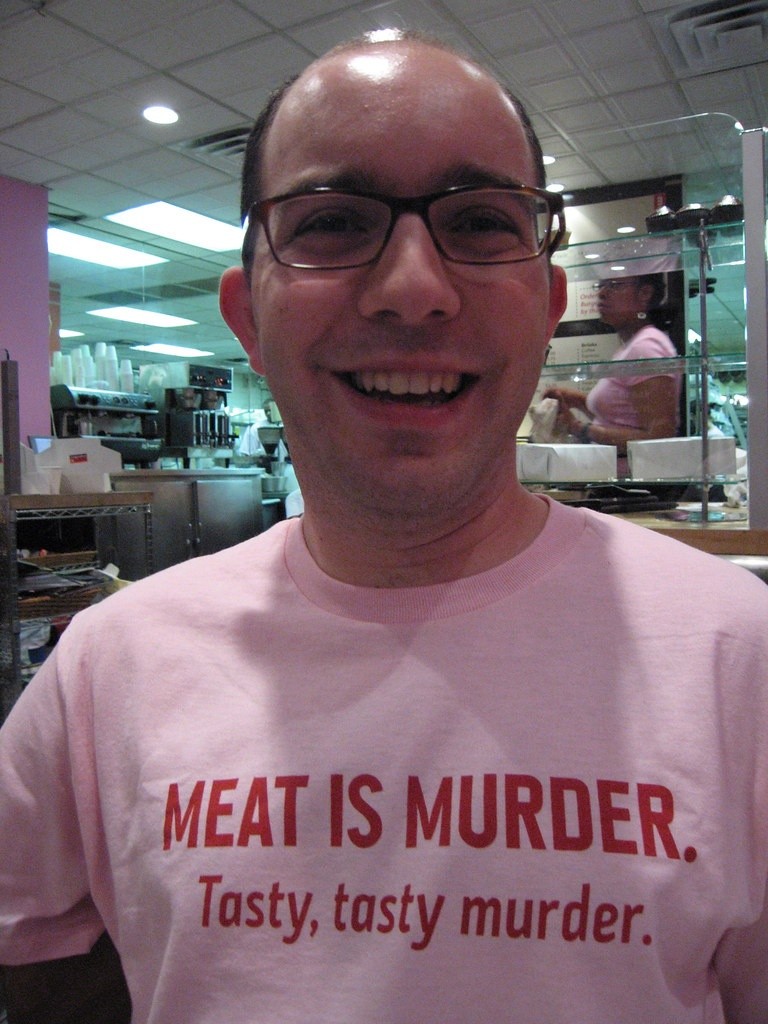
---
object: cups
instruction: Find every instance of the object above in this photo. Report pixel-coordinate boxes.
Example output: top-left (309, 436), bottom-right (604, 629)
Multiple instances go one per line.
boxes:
top-left (50, 342), bottom-right (135, 393)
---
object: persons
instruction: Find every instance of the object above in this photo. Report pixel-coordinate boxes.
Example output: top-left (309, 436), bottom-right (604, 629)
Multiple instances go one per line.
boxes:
top-left (541, 273), bottom-right (682, 476)
top-left (0, 39), bottom-right (768, 1024)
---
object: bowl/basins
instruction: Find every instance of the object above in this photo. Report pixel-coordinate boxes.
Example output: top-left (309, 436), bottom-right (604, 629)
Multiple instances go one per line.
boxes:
top-left (646, 194), bottom-right (744, 233)
top-left (546, 229), bottom-right (571, 251)
top-left (261, 476), bottom-right (288, 492)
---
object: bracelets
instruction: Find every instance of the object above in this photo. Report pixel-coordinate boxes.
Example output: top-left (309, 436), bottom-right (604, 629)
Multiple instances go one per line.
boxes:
top-left (580, 423), bottom-right (592, 444)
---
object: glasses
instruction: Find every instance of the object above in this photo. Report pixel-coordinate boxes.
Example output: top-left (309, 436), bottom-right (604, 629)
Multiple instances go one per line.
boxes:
top-left (241, 182), bottom-right (566, 273)
top-left (592, 276), bottom-right (643, 295)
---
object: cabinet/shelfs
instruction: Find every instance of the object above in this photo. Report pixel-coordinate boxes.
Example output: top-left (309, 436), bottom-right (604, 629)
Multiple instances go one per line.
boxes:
top-left (513, 217), bottom-right (749, 487)
top-left (109, 475), bottom-right (262, 583)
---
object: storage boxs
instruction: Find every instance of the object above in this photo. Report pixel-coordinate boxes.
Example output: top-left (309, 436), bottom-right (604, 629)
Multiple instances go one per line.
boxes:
top-left (625, 435), bottom-right (736, 478)
top-left (514, 443), bottom-right (618, 481)
